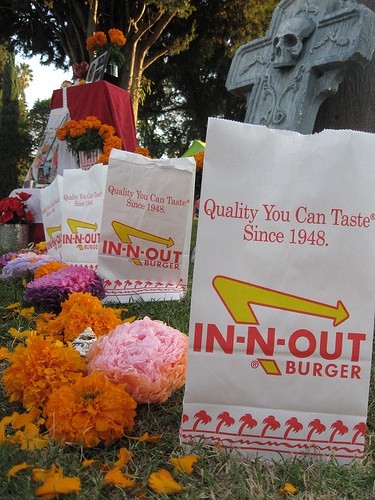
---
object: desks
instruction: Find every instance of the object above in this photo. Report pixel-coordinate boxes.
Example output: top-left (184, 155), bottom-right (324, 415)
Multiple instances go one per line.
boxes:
top-left (51, 81), bottom-right (137, 153)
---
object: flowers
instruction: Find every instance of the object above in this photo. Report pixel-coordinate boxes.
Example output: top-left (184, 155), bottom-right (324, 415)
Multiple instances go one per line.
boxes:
top-left (189, 150), bottom-right (204, 172)
top-left (57, 116), bottom-right (122, 165)
top-left (135, 147), bottom-right (156, 159)
top-left (0, 191), bottom-right (35, 226)
top-left (86, 29), bottom-right (126, 72)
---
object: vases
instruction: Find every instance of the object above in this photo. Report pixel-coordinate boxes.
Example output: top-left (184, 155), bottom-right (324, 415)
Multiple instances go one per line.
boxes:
top-left (0, 224), bottom-right (29, 256)
top-left (106, 60), bottom-right (119, 78)
top-left (79, 149), bottom-right (103, 171)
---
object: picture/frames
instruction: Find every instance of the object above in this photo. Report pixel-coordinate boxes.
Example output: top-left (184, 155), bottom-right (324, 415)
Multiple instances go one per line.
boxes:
top-left (86, 50), bottom-right (109, 84)
